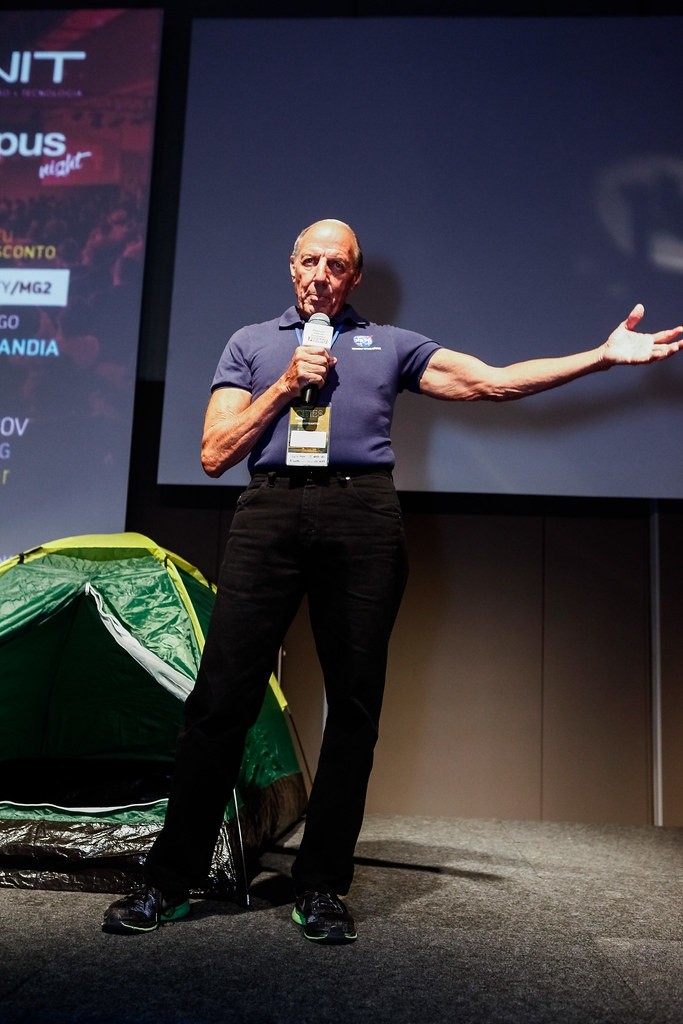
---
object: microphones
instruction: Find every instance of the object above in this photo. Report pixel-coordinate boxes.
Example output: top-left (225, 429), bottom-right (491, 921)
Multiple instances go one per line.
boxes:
top-left (301, 312), bottom-right (335, 404)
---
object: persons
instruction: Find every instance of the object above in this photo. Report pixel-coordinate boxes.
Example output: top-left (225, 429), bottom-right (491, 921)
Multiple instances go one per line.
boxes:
top-left (103, 218), bottom-right (683, 940)
top-left (0, 179), bottom-right (147, 476)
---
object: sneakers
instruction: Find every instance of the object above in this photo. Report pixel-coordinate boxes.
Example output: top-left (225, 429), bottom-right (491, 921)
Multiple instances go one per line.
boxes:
top-left (102, 881), bottom-right (190, 935)
top-left (292, 890), bottom-right (358, 940)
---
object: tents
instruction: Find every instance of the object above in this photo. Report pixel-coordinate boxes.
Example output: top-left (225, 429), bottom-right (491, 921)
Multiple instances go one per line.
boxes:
top-left (0, 533), bottom-right (314, 912)
